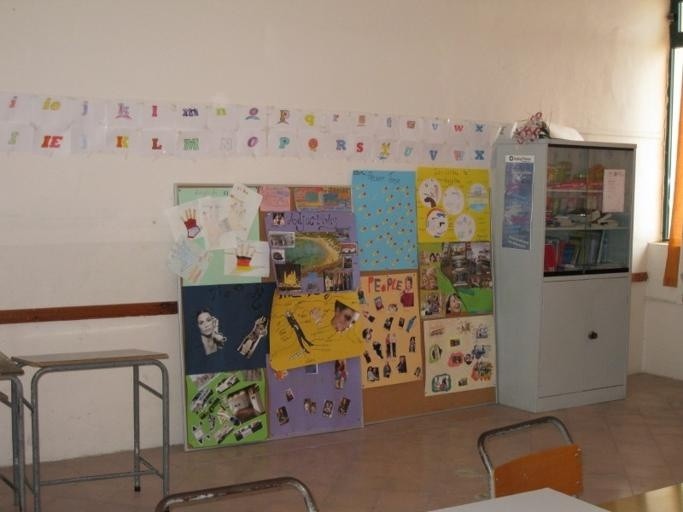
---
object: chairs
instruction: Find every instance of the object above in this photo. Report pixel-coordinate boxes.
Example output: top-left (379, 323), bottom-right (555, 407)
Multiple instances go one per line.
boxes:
top-left (474, 414), bottom-right (589, 498)
top-left (153, 476), bottom-right (320, 510)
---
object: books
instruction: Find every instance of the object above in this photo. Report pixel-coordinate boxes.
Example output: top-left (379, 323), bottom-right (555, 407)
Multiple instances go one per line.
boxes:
top-left (545, 231), bottom-right (612, 273)
top-left (547, 201), bottom-right (617, 231)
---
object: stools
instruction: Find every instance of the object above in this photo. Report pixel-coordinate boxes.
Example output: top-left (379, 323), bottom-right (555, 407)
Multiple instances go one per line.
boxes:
top-left (1, 350), bottom-right (24, 511)
top-left (15, 351), bottom-right (169, 509)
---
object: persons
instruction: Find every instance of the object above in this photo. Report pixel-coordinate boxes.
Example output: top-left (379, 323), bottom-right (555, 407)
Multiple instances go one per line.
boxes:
top-left (400, 276), bottom-right (414, 308)
top-left (196, 311), bottom-right (228, 357)
top-left (445, 293), bottom-right (461, 314)
top-left (330, 299), bottom-right (360, 334)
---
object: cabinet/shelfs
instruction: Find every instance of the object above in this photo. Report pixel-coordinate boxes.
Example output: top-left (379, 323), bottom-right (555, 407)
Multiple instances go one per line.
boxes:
top-left (492, 138), bottom-right (639, 414)
